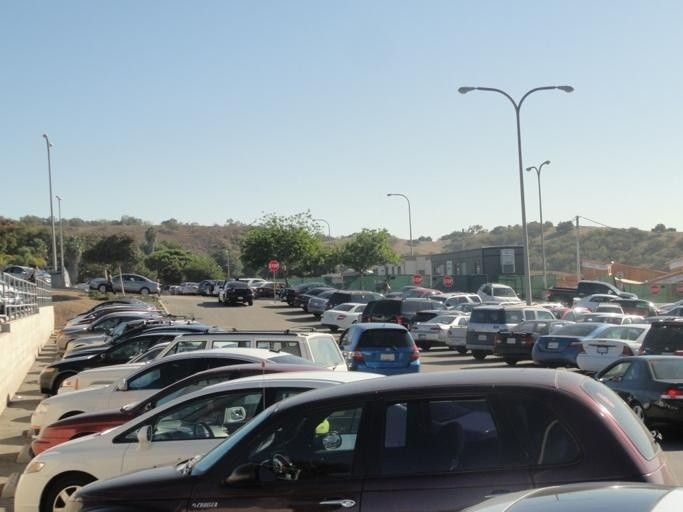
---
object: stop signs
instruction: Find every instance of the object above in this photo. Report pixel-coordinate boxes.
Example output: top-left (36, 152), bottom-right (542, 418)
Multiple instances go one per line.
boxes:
top-left (268, 260), bottom-right (278, 272)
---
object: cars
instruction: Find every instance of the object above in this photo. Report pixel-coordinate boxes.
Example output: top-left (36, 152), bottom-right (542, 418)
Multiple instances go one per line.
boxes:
top-left (575, 324), bottom-right (652, 373)
top-left (493, 318), bottom-right (577, 366)
top-left (532, 321), bottom-right (610, 370)
top-left (459, 480), bottom-right (683, 512)
top-left (586, 354), bottom-right (683, 433)
top-left (445, 324), bottom-right (466, 355)
top-left (30, 296), bottom-right (349, 455)
top-left (336, 323), bottom-right (421, 375)
top-left (279, 278), bottom-right (683, 332)
top-left (408, 314), bottom-right (470, 351)
top-left (12, 369), bottom-right (387, 512)
top-left (0, 265), bottom-right (51, 313)
top-left (167, 277), bottom-right (290, 306)
top-left (88, 273), bottom-right (160, 295)
top-left (63, 370), bottom-right (675, 511)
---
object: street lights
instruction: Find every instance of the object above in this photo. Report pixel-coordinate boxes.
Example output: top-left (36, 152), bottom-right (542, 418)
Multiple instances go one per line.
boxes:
top-left (386, 193), bottom-right (413, 256)
top-left (311, 219), bottom-right (330, 245)
top-left (54, 195), bottom-right (65, 275)
top-left (457, 85), bottom-right (575, 305)
top-left (42, 133), bottom-right (57, 274)
top-left (525, 160), bottom-right (553, 292)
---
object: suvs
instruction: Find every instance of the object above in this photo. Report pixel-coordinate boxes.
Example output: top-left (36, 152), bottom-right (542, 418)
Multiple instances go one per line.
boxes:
top-left (637, 321), bottom-right (683, 357)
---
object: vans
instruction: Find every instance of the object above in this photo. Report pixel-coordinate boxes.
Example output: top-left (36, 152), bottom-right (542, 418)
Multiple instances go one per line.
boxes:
top-left (464, 305), bottom-right (558, 361)
